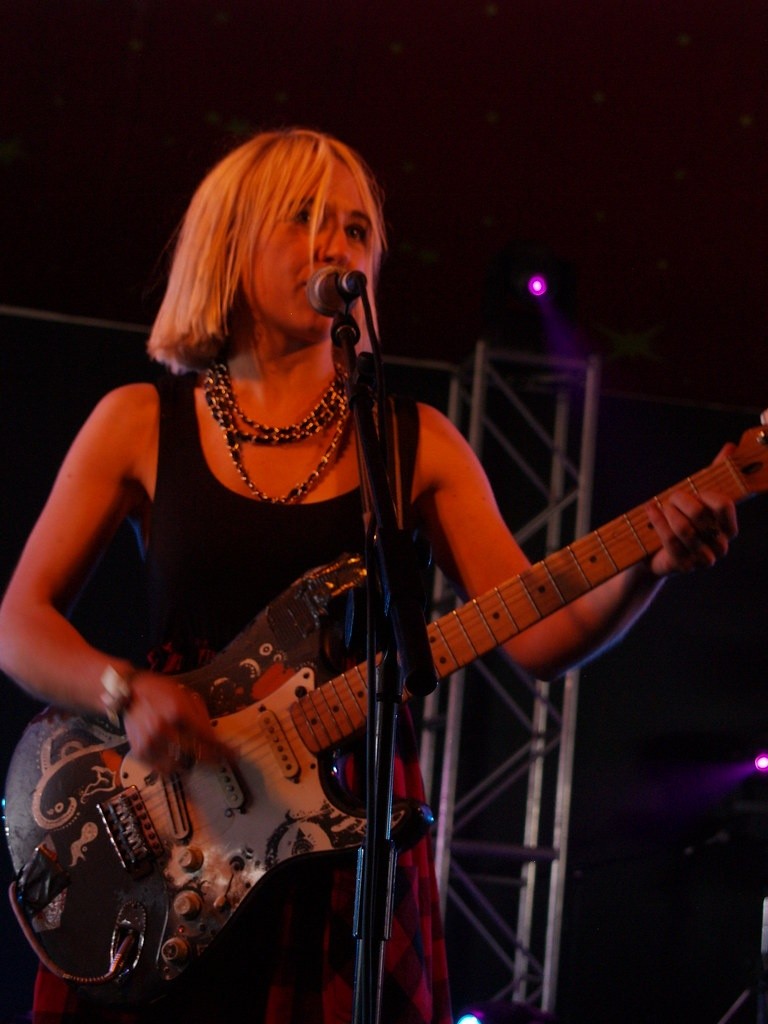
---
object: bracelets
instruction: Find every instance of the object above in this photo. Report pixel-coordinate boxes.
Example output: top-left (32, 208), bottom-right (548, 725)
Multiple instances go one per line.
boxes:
top-left (100, 661), bottom-right (134, 727)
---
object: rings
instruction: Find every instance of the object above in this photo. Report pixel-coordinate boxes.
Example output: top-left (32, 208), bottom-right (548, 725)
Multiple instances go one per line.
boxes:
top-left (701, 525), bottom-right (722, 543)
top-left (671, 550), bottom-right (710, 589)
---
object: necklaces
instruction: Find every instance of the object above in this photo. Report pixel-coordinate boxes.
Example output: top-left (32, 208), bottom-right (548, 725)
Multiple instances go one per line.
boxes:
top-left (200, 349), bottom-right (367, 509)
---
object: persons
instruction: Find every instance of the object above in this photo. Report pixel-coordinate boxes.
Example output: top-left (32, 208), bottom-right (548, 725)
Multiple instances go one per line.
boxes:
top-left (0, 120), bottom-right (742, 1024)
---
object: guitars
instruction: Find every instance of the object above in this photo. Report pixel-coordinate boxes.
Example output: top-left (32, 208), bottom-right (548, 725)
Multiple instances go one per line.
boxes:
top-left (5, 408), bottom-right (768, 992)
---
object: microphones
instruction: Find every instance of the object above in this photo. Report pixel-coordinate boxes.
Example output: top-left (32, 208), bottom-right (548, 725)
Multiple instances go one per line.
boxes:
top-left (304, 266), bottom-right (371, 317)
